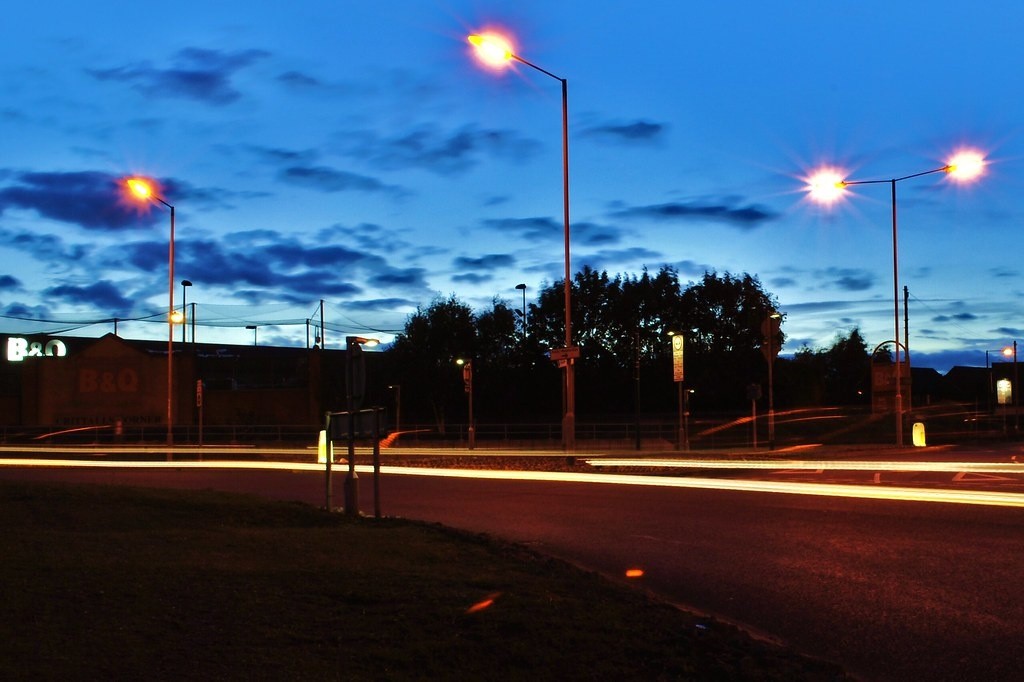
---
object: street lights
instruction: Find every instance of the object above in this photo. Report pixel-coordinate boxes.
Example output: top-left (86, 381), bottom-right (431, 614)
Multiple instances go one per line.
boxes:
top-left (516, 283), bottom-right (526, 340)
top-left (986, 347), bottom-right (1012, 414)
top-left (126, 177), bottom-right (176, 462)
top-left (467, 30), bottom-right (576, 452)
top-left (247, 325), bottom-right (260, 345)
top-left (806, 149), bottom-right (984, 447)
top-left (455, 358), bottom-right (474, 450)
top-left (180, 281), bottom-right (193, 342)
top-left (766, 308), bottom-right (780, 450)
top-left (667, 328), bottom-right (698, 452)
top-left (388, 384), bottom-right (400, 446)
